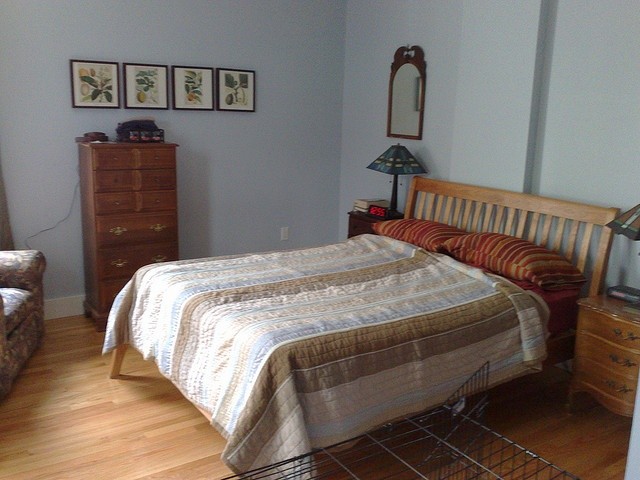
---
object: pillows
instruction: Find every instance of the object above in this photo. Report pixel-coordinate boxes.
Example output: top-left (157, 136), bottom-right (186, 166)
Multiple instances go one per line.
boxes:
top-left (434, 232), bottom-right (588, 288)
top-left (369, 218), bottom-right (466, 255)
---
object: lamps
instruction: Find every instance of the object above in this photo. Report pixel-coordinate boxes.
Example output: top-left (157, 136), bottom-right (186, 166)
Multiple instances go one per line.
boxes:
top-left (604, 201), bottom-right (639, 305)
top-left (366, 144), bottom-right (426, 219)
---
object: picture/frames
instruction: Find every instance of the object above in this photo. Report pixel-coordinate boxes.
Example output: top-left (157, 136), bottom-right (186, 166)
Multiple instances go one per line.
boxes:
top-left (70, 58), bottom-right (120, 109)
top-left (171, 66), bottom-right (215, 110)
top-left (216, 67), bottom-right (256, 112)
top-left (123, 62), bottom-right (169, 109)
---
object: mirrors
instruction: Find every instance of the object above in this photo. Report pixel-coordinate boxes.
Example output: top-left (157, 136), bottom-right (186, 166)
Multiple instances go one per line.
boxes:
top-left (387, 43), bottom-right (427, 139)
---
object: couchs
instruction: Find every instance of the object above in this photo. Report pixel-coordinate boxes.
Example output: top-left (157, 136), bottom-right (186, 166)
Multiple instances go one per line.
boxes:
top-left (1, 249), bottom-right (47, 397)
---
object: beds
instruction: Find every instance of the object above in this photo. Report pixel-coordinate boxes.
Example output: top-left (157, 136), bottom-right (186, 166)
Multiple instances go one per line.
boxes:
top-left (102, 178), bottom-right (621, 480)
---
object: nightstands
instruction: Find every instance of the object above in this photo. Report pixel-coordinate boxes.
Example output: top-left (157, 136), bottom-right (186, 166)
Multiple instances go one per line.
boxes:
top-left (346, 208), bottom-right (421, 239)
top-left (565, 294), bottom-right (640, 417)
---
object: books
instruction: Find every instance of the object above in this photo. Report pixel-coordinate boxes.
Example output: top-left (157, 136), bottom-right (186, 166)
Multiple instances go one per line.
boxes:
top-left (352, 197), bottom-right (390, 215)
top-left (606, 285), bottom-right (640, 305)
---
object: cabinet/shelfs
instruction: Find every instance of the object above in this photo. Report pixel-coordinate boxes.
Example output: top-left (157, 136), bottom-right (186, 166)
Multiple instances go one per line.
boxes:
top-left (76, 137), bottom-right (180, 332)
